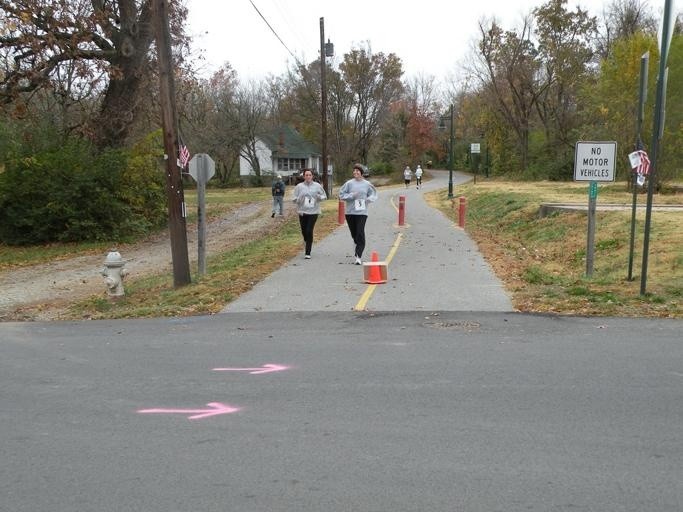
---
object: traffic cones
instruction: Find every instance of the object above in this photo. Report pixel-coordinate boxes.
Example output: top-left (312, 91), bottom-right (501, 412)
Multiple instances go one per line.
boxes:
top-left (365, 252), bottom-right (386, 284)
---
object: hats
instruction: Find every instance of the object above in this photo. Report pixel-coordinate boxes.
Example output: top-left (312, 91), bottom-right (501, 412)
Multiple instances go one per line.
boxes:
top-left (353, 163), bottom-right (365, 176)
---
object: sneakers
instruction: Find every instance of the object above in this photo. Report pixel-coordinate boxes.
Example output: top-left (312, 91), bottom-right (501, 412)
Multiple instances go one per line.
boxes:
top-left (306, 249), bottom-right (310, 259)
top-left (354, 255), bottom-right (361, 264)
top-left (271, 212), bottom-right (275, 218)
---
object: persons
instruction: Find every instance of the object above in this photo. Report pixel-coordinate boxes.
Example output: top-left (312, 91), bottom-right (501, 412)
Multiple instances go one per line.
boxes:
top-left (415, 165), bottom-right (423, 189)
top-left (291, 169), bottom-right (326, 259)
top-left (403, 166), bottom-right (411, 189)
top-left (337, 164), bottom-right (377, 264)
top-left (270, 174), bottom-right (285, 217)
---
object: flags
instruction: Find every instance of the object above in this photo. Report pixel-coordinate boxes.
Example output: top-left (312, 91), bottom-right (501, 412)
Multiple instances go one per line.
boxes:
top-left (177, 137), bottom-right (188, 167)
top-left (635, 136), bottom-right (650, 177)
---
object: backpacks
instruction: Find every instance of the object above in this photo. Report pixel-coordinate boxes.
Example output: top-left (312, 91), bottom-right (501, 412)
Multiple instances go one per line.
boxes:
top-left (273, 181), bottom-right (282, 196)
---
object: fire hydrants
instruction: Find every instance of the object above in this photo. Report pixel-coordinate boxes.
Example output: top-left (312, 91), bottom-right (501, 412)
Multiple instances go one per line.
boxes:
top-left (101, 244), bottom-right (128, 296)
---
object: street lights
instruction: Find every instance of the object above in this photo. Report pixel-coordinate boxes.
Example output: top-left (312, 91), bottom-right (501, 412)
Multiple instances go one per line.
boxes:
top-left (438, 104), bottom-right (454, 198)
